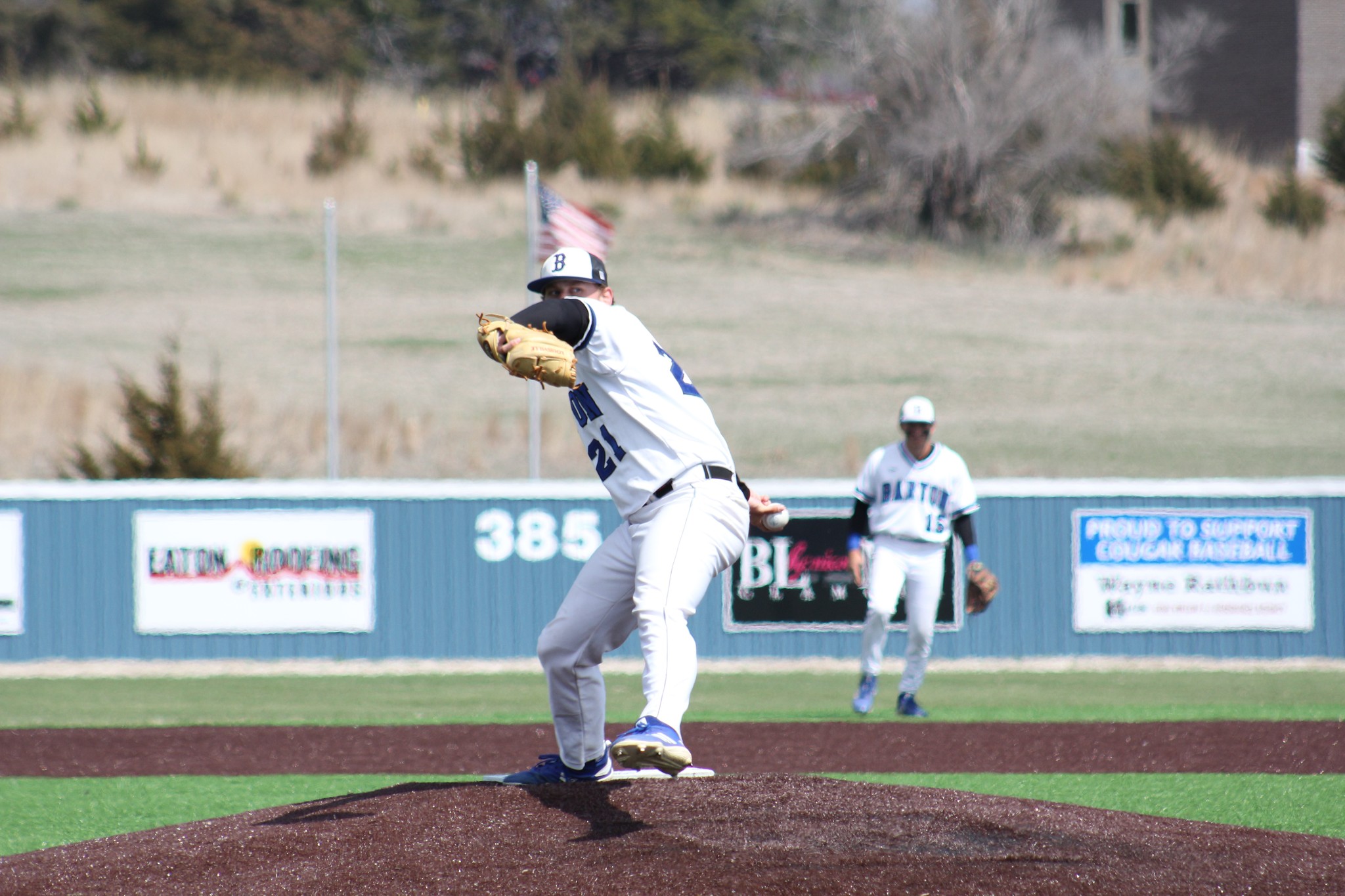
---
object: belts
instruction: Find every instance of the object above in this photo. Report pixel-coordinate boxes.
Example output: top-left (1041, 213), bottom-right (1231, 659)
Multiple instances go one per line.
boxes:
top-left (642, 466), bottom-right (732, 507)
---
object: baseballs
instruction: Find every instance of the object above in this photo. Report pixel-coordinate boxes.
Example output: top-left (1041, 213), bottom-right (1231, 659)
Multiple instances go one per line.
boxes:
top-left (763, 503), bottom-right (790, 530)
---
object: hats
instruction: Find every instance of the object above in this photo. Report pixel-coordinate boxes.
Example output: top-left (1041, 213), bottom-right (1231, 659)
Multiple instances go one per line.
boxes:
top-left (527, 247), bottom-right (608, 294)
top-left (900, 395), bottom-right (935, 424)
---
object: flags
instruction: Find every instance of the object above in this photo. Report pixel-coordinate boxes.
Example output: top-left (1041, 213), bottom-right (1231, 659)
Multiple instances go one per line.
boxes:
top-left (538, 182), bottom-right (616, 271)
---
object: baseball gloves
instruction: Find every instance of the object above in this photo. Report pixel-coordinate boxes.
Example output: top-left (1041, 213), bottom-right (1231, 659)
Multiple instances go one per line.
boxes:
top-left (476, 313), bottom-right (578, 389)
top-left (965, 561), bottom-right (999, 614)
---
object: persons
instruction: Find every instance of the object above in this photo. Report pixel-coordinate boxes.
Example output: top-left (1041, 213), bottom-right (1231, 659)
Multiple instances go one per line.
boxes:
top-left (476, 247), bottom-right (785, 785)
top-left (848, 396), bottom-right (998, 716)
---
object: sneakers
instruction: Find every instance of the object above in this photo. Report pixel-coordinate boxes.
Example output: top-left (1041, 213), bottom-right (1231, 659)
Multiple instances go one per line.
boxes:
top-left (853, 675), bottom-right (878, 714)
top-left (896, 691), bottom-right (927, 717)
top-left (612, 715), bottom-right (692, 781)
top-left (502, 740), bottom-right (614, 784)
